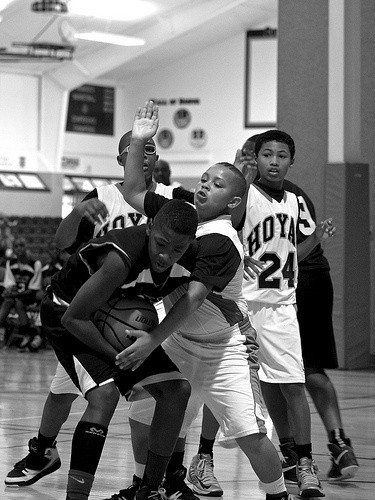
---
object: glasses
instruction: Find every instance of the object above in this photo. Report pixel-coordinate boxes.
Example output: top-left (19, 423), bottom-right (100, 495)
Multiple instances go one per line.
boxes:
top-left (120, 144), bottom-right (156, 156)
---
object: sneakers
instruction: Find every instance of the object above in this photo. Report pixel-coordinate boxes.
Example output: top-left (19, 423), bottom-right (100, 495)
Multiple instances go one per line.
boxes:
top-left (296, 457), bottom-right (325, 498)
top-left (4, 437), bottom-right (61, 488)
top-left (103, 479), bottom-right (201, 500)
top-left (327, 437), bottom-right (360, 481)
top-left (279, 455), bottom-right (300, 484)
top-left (184, 454), bottom-right (223, 496)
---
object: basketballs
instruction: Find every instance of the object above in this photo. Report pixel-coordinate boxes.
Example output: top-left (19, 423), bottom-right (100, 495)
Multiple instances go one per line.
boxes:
top-left (92, 296), bottom-right (160, 353)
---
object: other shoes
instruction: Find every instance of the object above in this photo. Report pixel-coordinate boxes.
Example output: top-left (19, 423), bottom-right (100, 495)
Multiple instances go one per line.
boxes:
top-left (25, 335), bottom-right (42, 351)
top-left (17, 336), bottom-right (30, 352)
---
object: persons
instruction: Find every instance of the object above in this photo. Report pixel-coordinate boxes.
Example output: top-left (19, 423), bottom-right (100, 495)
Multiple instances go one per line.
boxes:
top-left (0, 101), bottom-right (360, 500)
